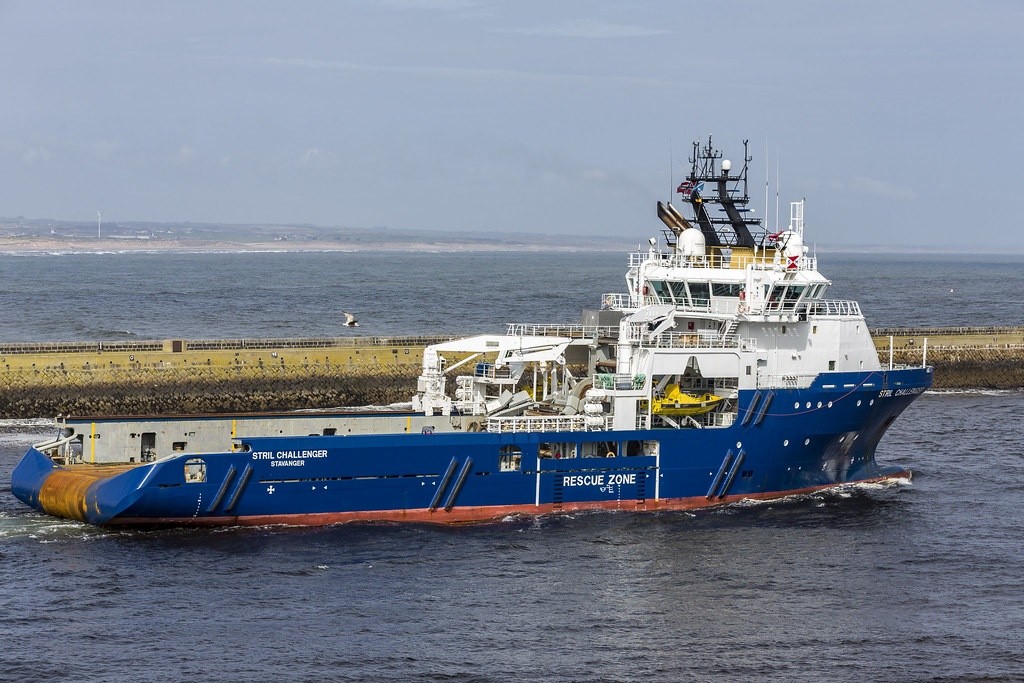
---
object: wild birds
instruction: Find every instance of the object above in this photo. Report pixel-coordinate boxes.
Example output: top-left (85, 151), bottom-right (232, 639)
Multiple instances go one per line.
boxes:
top-left (339, 308), bottom-right (360, 328)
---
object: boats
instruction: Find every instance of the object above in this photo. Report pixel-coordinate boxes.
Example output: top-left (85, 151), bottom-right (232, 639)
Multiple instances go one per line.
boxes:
top-left (11, 135), bottom-right (934, 527)
